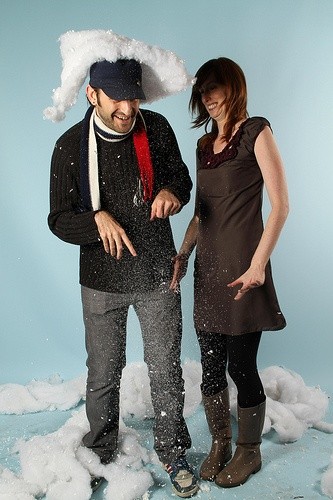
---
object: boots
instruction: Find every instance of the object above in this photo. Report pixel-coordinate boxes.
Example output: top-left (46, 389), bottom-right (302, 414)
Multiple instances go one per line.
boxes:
top-left (214, 395), bottom-right (267, 488)
top-left (199, 383), bottom-right (233, 482)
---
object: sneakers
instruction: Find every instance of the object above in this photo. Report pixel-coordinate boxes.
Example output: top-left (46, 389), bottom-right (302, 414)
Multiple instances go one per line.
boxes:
top-left (162, 456), bottom-right (199, 498)
top-left (90, 462), bottom-right (108, 492)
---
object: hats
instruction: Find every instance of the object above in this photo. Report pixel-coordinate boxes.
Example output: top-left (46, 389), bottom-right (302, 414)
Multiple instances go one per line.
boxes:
top-left (90, 58), bottom-right (147, 100)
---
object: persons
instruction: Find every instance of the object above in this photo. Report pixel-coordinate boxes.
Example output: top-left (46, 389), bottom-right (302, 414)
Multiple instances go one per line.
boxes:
top-left (47, 59), bottom-right (200, 498)
top-left (169, 57), bottom-right (289, 489)
